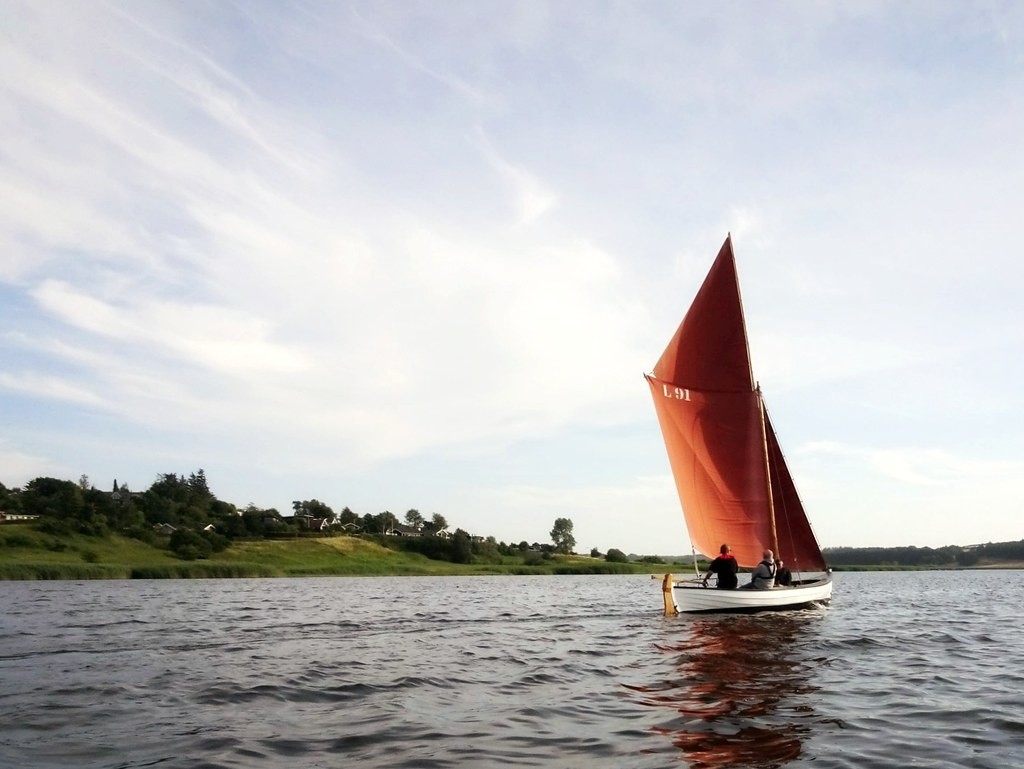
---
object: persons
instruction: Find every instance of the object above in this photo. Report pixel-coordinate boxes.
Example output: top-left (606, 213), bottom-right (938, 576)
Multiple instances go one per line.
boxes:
top-left (739, 549), bottom-right (777, 590)
top-left (775, 560), bottom-right (792, 586)
top-left (703, 544), bottom-right (738, 590)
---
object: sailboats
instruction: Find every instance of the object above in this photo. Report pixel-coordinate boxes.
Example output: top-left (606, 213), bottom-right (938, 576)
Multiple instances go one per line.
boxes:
top-left (643, 230), bottom-right (834, 616)
